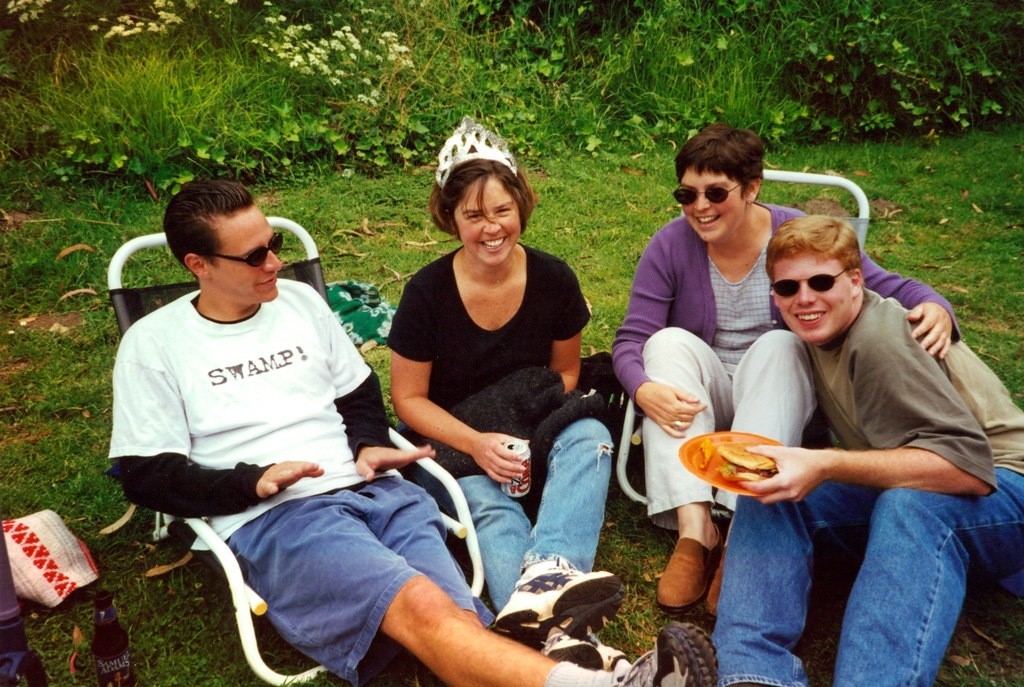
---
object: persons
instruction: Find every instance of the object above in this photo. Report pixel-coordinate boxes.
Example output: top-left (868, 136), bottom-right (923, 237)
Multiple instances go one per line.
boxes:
top-left (107, 180), bottom-right (716, 687)
top-left (610, 123), bottom-right (961, 613)
top-left (712, 214), bottom-right (1024, 687)
top-left (388, 114), bottom-right (623, 672)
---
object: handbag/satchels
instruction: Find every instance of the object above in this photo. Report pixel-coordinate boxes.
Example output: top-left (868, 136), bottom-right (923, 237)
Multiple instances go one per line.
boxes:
top-left (3, 508), bottom-right (101, 607)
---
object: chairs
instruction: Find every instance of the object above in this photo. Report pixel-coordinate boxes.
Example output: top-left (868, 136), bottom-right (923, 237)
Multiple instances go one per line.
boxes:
top-left (615, 166), bottom-right (871, 519)
top-left (108, 216), bottom-right (487, 687)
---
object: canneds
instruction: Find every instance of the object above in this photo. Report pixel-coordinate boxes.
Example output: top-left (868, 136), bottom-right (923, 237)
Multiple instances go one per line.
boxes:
top-left (500, 439), bottom-right (532, 497)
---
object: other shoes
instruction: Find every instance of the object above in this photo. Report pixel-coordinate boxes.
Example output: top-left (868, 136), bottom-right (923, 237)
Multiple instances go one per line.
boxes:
top-left (702, 539), bottom-right (730, 614)
top-left (656, 522), bottom-right (722, 614)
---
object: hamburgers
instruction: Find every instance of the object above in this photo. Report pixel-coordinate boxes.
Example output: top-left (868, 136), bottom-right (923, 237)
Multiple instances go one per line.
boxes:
top-left (718, 442), bottom-right (779, 482)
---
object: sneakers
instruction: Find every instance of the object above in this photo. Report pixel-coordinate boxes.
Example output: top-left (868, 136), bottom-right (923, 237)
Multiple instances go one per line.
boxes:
top-left (495, 556), bottom-right (625, 649)
top-left (539, 631), bottom-right (605, 672)
top-left (613, 622), bottom-right (719, 687)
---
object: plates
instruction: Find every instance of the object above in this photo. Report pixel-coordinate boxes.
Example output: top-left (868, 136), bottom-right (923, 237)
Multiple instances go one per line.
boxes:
top-left (679, 431), bottom-right (783, 497)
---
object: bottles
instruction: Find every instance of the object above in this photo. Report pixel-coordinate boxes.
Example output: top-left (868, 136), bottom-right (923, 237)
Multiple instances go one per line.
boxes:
top-left (91, 589), bottom-right (133, 687)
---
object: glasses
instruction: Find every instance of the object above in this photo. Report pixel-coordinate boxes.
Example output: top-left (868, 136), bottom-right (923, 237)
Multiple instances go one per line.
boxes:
top-left (768, 268), bottom-right (848, 296)
top-left (672, 183), bottom-right (742, 205)
top-left (192, 231), bottom-right (285, 267)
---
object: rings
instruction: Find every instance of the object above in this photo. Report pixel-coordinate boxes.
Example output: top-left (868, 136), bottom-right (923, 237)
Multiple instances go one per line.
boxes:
top-left (675, 421), bottom-right (681, 429)
